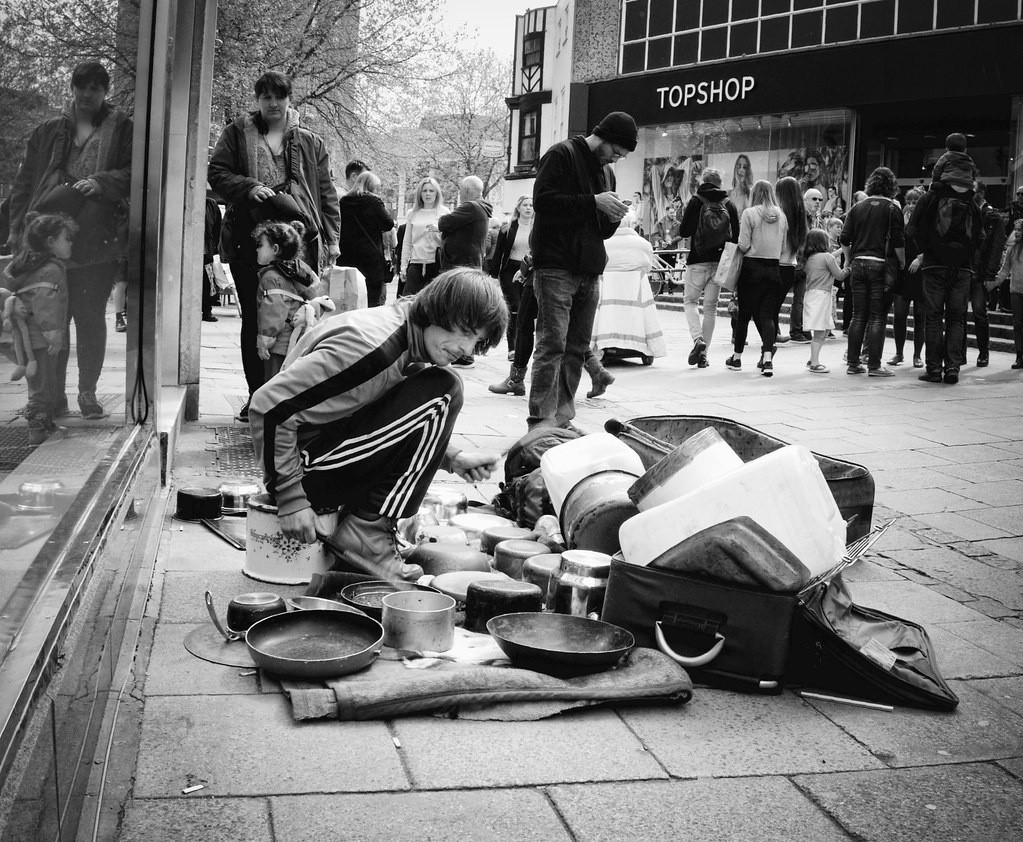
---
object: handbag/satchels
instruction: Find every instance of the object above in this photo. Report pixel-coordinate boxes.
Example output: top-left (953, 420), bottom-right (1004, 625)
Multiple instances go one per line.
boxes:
top-left (211, 253), bottom-right (239, 290)
top-left (380, 253), bottom-right (394, 283)
top-left (318, 254), bottom-right (368, 321)
top-left (251, 179), bottom-right (320, 243)
top-left (31, 172), bottom-right (116, 266)
top-left (714, 241), bottom-right (743, 293)
top-left (884, 256), bottom-right (909, 297)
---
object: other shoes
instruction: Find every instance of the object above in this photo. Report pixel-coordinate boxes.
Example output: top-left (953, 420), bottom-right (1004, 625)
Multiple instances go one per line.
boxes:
top-left (669, 287), bottom-right (673, 294)
top-left (450, 350), bottom-right (515, 367)
top-left (115, 312), bottom-right (128, 331)
top-left (27, 424), bottom-right (69, 444)
top-left (688, 325), bottom-right (1023, 383)
top-left (203, 311), bottom-right (219, 322)
top-left (558, 422), bottom-right (587, 435)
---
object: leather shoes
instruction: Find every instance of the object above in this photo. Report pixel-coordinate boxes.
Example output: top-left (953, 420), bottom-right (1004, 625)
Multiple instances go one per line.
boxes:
top-left (330, 512), bottom-right (426, 582)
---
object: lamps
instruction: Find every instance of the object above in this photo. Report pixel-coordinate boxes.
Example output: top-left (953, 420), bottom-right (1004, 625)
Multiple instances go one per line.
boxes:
top-left (786, 118), bottom-right (794, 128)
top-left (736, 123), bottom-right (744, 132)
top-left (756, 120), bottom-right (764, 130)
top-left (660, 130), bottom-right (669, 138)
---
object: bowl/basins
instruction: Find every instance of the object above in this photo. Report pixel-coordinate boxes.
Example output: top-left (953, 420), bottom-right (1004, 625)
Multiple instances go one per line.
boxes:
top-left (215, 481), bottom-right (262, 515)
top-left (244, 609), bottom-right (385, 685)
top-left (446, 513), bottom-right (514, 546)
top-left (485, 612), bottom-right (635, 682)
top-left (285, 595), bottom-right (367, 616)
top-left (339, 580), bottom-right (443, 624)
top-left (492, 540), bottom-right (551, 580)
top-left (403, 543), bottom-right (490, 577)
top-left (396, 506), bottom-right (439, 545)
top-left (463, 580), bottom-right (543, 635)
top-left (522, 553), bottom-right (562, 605)
top-left (558, 469), bottom-right (640, 556)
top-left (416, 525), bottom-right (469, 546)
top-left (480, 527), bottom-right (537, 557)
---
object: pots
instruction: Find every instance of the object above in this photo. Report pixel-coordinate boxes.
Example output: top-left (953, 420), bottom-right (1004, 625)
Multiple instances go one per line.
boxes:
top-left (422, 489), bottom-right (493, 522)
top-left (204, 590), bottom-right (287, 642)
top-left (172, 486), bottom-right (246, 550)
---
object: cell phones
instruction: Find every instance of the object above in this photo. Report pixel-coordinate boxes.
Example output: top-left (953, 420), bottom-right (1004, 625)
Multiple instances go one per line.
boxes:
top-left (622, 200), bottom-right (632, 206)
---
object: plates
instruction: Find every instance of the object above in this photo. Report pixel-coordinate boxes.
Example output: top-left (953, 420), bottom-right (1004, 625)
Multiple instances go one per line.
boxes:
top-left (432, 571), bottom-right (511, 602)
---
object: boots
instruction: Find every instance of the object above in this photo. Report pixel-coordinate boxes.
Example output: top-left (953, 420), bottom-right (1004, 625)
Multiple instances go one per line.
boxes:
top-left (584, 356), bottom-right (616, 399)
top-left (488, 363), bottom-right (527, 395)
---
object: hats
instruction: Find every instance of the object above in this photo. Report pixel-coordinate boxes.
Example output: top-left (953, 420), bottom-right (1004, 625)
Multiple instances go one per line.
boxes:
top-left (594, 111), bottom-right (638, 152)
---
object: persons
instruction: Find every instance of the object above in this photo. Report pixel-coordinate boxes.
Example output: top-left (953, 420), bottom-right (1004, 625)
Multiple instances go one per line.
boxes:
top-left (651, 157), bottom-right (691, 224)
top-left (1, 60), bottom-right (135, 427)
top-left (725, 152), bottom-right (755, 219)
top-left (208, 72), bottom-right (1023, 435)
top-left (799, 148), bottom-right (828, 210)
top-left (248, 264), bottom-right (502, 583)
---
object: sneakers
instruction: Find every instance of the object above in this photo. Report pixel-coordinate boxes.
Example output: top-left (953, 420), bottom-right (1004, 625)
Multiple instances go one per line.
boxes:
top-left (78, 388), bottom-right (106, 420)
top-left (239, 399), bottom-right (250, 420)
top-left (54, 394), bottom-right (69, 415)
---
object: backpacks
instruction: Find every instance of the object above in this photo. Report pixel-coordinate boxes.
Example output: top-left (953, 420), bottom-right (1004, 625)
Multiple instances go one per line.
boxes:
top-left (691, 192), bottom-right (732, 256)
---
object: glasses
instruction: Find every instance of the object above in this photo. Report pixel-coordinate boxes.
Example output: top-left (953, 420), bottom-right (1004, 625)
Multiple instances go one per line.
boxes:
top-left (610, 142), bottom-right (628, 161)
top-left (1016, 192), bottom-right (1023, 197)
top-left (808, 197), bottom-right (824, 203)
